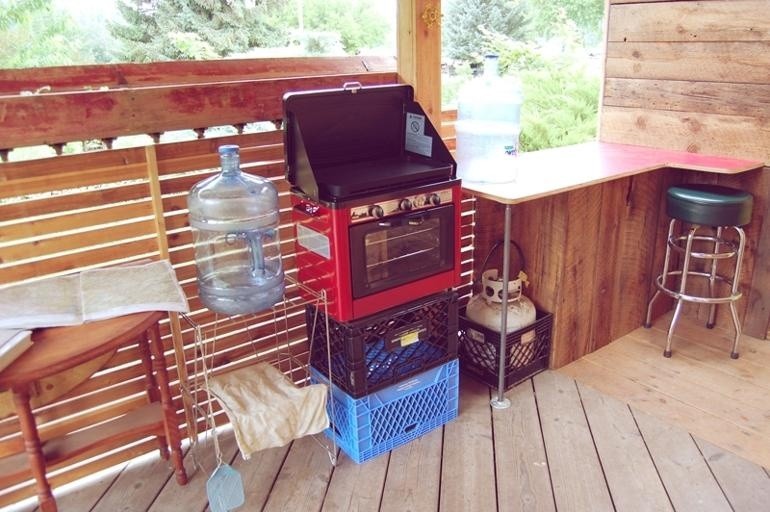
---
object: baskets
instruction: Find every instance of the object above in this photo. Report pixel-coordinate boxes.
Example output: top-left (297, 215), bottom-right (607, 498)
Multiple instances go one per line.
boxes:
top-left (304, 292), bottom-right (553, 465)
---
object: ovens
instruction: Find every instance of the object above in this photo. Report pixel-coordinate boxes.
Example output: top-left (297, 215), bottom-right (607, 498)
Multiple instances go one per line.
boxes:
top-left (290, 177), bottom-right (462, 323)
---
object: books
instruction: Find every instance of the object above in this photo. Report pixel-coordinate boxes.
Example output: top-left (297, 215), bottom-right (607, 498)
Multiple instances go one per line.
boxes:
top-left (0, 258), bottom-right (190, 375)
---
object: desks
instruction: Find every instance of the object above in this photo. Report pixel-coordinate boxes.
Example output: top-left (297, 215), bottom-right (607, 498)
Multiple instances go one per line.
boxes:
top-left (0, 257), bottom-right (185, 511)
top-left (459, 139), bottom-right (764, 411)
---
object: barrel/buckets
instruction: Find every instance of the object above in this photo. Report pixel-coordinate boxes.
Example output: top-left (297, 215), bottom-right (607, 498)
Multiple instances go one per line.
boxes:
top-left (454, 52), bottom-right (523, 184)
top-left (185, 144), bottom-right (283, 315)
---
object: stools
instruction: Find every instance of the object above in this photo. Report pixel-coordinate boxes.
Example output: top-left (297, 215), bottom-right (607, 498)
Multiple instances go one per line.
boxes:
top-left (644, 184), bottom-right (754, 360)
